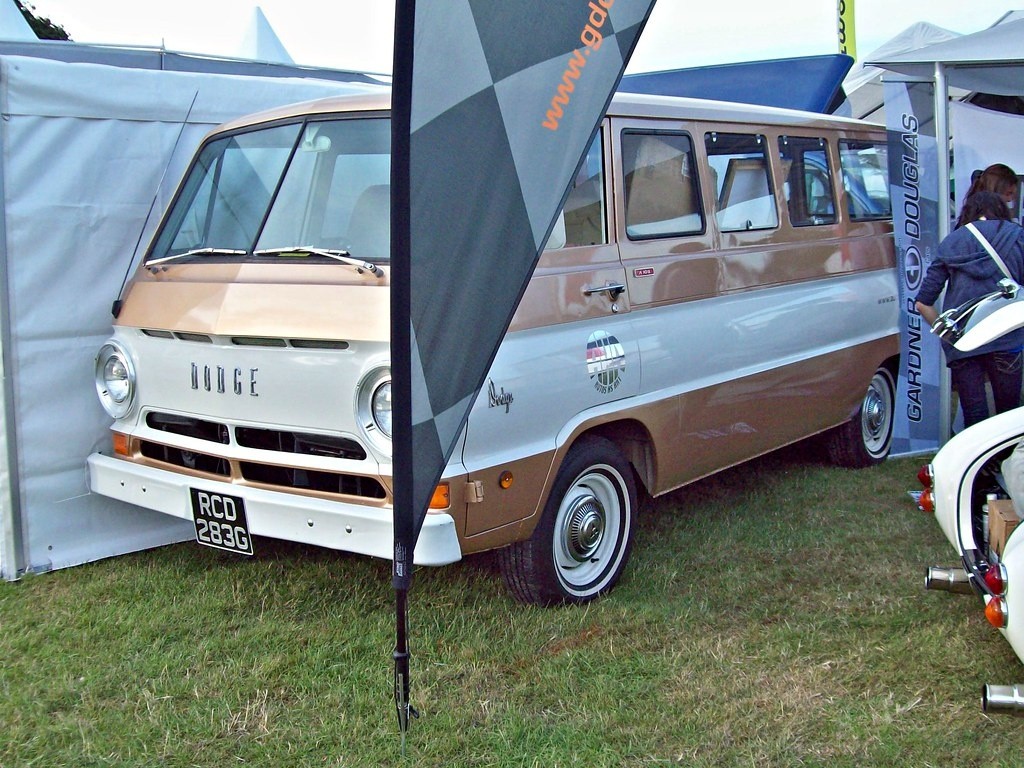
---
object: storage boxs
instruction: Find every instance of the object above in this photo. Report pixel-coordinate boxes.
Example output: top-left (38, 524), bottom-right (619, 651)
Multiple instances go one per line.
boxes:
top-left (562, 134), bottom-right (719, 247)
top-left (987, 499), bottom-right (1022, 564)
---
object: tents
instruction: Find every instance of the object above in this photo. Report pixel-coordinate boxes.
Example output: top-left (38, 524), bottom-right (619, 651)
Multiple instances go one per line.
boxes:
top-left (0, 4), bottom-right (1024, 593)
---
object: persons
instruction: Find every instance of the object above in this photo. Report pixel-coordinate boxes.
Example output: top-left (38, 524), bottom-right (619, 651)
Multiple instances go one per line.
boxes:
top-left (913, 190), bottom-right (1024, 431)
top-left (964, 163), bottom-right (1018, 205)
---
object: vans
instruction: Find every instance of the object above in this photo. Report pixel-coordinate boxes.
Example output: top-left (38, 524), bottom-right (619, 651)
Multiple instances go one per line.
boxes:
top-left (87, 92), bottom-right (902, 607)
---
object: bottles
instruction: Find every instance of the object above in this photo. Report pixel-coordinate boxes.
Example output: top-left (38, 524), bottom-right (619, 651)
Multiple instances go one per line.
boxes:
top-left (983, 494), bottom-right (997, 543)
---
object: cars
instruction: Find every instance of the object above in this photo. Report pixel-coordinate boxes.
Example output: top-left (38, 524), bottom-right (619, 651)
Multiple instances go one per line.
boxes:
top-left (916, 278), bottom-right (1024, 721)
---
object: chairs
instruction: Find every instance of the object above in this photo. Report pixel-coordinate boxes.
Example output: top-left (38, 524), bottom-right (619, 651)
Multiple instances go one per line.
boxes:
top-left (348, 185), bottom-right (397, 267)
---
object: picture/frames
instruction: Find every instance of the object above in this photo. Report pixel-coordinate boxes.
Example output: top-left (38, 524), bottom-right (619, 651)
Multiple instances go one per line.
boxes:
top-left (718, 156), bottom-right (794, 230)
